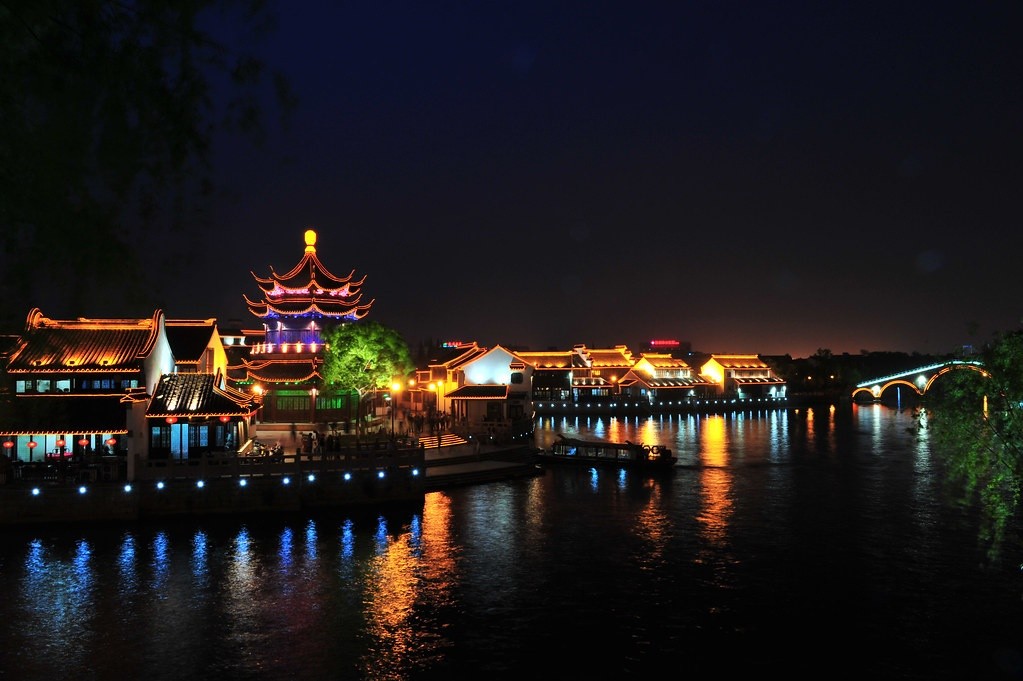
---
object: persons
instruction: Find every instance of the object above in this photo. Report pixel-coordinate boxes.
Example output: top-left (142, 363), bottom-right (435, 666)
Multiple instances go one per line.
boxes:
top-left (386, 404), bottom-right (392, 421)
top-left (289, 416), bottom-right (350, 460)
top-left (397, 408), bottom-right (529, 450)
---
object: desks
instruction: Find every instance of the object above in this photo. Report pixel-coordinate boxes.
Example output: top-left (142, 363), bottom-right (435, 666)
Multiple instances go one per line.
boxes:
top-left (46, 452), bottom-right (73, 462)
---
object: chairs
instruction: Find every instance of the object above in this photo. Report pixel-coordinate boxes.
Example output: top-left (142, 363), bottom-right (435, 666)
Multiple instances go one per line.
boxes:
top-left (45, 448), bottom-right (71, 464)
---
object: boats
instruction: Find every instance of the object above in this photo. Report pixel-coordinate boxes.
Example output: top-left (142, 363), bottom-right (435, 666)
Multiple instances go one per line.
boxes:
top-left (539, 431), bottom-right (678, 470)
